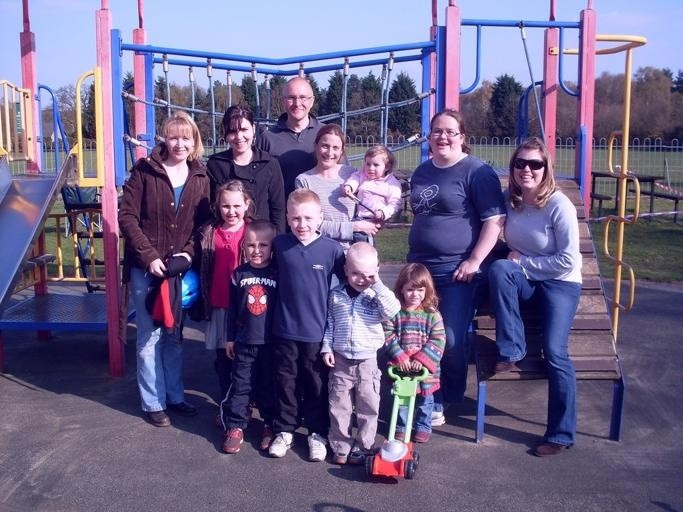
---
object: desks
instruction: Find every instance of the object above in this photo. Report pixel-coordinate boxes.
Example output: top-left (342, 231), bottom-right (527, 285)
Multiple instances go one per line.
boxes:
top-left (392, 168), bottom-right (412, 212)
top-left (590, 171), bottom-right (664, 224)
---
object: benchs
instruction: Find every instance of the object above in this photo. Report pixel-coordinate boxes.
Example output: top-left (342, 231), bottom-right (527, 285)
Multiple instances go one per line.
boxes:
top-left (590, 191), bottom-right (611, 219)
top-left (629, 187), bottom-right (682, 224)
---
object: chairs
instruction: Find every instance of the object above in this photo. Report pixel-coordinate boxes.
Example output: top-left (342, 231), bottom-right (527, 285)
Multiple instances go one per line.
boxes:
top-left (61, 180), bottom-right (103, 236)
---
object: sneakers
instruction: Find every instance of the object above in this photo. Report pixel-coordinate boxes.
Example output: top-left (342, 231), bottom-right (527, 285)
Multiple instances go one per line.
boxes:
top-left (307, 433), bottom-right (326, 462)
top-left (222, 427), bottom-right (244, 454)
top-left (260, 431), bottom-right (295, 458)
top-left (350, 452), bottom-right (366, 463)
top-left (332, 452), bottom-right (348, 464)
top-left (413, 431), bottom-right (430, 442)
top-left (430, 412), bottom-right (445, 427)
top-left (394, 432), bottom-right (405, 443)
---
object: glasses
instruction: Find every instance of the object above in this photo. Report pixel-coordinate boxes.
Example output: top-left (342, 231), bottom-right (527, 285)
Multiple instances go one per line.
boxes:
top-left (512, 158), bottom-right (544, 170)
top-left (432, 128), bottom-right (460, 137)
top-left (284, 95), bottom-right (312, 101)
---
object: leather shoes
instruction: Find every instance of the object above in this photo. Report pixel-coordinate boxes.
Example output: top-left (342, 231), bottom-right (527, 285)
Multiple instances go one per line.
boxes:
top-left (493, 360), bottom-right (517, 374)
top-left (534, 441), bottom-right (572, 456)
top-left (145, 411), bottom-right (171, 427)
top-left (167, 402), bottom-right (198, 416)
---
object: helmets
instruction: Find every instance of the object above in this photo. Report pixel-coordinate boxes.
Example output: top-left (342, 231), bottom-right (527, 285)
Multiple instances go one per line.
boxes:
top-left (180, 269), bottom-right (200, 308)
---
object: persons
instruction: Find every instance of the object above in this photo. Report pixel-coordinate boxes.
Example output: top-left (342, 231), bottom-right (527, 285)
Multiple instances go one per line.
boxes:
top-left (319, 241), bottom-right (402, 464)
top-left (403, 109), bottom-right (506, 427)
top-left (206, 104), bottom-right (286, 233)
top-left (197, 180), bottom-right (259, 428)
top-left (484, 136), bottom-right (583, 456)
top-left (268, 188), bottom-right (350, 462)
top-left (118, 112), bottom-right (211, 426)
top-left (382, 263), bottom-right (446, 442)
top-left (342, 145), bottom-right (403, 245)
top-left (222, 218), bottom-right (282, 455)
top-left (255, 77), bottom-right (327, 196)
